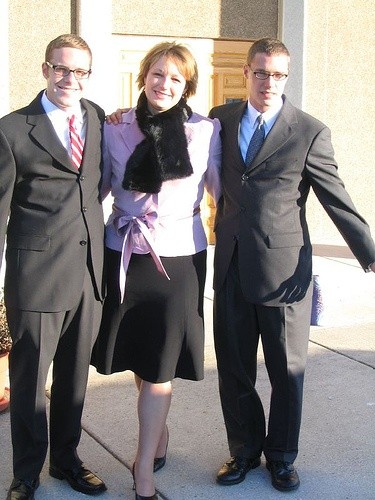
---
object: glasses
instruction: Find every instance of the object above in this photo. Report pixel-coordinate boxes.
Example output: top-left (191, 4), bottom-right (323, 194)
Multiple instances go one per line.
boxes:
top-left (248, 63), bottom-right (288, 81)
top-left (46, 61), bottom-right (92, 79)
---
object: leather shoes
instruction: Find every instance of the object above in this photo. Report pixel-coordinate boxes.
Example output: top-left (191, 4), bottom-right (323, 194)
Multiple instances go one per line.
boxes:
top-left (217, 456), bottom-right (261, 484)
top-left (6, 476), bottom-right (40, 500)
top-left (266, 460), bottom-right (300, 490)
top-left (49, 464), bottom-right (105, 495)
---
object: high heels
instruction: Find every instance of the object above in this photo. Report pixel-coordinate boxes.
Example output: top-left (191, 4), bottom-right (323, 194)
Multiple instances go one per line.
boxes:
top-left (132, 462), bottom-right (157, 500)
top-left (153, 425), bottom-right (169, 472)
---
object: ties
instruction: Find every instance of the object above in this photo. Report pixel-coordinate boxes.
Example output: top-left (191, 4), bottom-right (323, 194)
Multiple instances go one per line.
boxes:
top-left (245, 115), bottom-right (265, 168)
top-left (68, 115), bottom-right (84, 171)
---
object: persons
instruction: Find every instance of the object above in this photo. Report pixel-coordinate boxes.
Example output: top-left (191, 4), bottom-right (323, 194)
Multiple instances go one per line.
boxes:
top-left (96, 41), bottom-right (226, 500)
top-left (0, 34), bottom-right (106, 500)
top-left (106, 37), bottom-right (375, 490)
